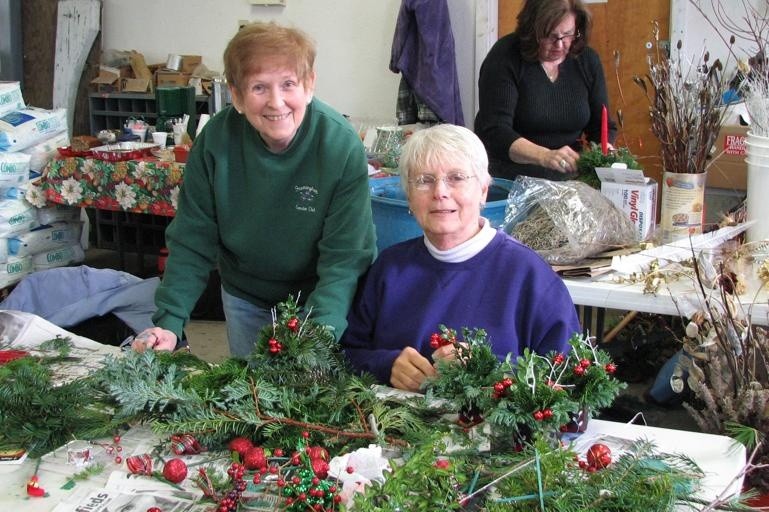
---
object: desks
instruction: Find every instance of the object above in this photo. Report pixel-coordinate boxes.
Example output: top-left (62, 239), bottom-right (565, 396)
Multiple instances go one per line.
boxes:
top-left (45, 138), bottom-right (769, 363)
top-left (0, 307), bottom-right (749, 512)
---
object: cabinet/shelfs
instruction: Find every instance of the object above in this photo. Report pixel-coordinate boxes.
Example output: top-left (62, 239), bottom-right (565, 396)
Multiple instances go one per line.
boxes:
top-left (88, 82), bottom-right (214, 253)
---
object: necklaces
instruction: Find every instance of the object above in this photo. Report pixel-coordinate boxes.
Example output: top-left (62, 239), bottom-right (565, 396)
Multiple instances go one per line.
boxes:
top-left (542, 60), bottom-right (560, 81)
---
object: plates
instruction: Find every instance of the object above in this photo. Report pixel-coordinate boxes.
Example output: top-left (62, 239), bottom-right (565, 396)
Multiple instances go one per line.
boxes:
top-left (55, 141), bottom-right (108, 158)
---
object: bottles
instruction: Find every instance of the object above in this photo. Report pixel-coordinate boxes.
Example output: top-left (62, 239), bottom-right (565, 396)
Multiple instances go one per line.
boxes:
top-left (209, 74), bottom-right (228, 120)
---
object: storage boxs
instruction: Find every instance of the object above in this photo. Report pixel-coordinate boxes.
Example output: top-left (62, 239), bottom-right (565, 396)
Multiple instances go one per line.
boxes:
top-left (595, 164), bottom-right (658, 244)
top-left (89, 48), bottom-right (214, 96)
top-left (367, 175), bottom-right (529, 264)
top-left (703, 126), bottom-right (748, 192)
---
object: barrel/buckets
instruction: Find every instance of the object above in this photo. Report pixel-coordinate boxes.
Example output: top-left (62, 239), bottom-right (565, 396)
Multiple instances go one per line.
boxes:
top-left (744, 129), bottom-right (769, 245)
top-left (369, 176), bottom-right (527, 253)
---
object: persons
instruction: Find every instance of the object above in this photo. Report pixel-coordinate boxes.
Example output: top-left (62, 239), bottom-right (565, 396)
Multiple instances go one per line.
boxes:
top-left (471, 1), bottom-right (620, 183)
top-left (337, 121), bottom-right (585, 399)
top-left (130, 19), bottom-right (380, 382)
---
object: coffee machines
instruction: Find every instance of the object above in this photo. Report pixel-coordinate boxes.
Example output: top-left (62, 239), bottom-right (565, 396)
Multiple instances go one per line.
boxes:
top-left (152, 84), bottom-right (196, 146)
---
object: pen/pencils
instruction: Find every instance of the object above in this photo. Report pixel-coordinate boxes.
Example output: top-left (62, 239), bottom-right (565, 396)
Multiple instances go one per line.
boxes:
top-left (174, 113), bottom-right (190, 124)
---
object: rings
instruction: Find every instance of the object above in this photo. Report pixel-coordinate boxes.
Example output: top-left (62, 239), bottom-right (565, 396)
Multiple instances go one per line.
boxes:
top-left (557, 159), bottom-right (566, 169)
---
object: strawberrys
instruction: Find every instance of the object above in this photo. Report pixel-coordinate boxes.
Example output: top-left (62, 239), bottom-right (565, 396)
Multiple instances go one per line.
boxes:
top-left (587, 444), bottom-right (610, 469)
top-left (163, 459), bottom-right (187, 482)
top-left (230, 439), bottom-right (328, 478)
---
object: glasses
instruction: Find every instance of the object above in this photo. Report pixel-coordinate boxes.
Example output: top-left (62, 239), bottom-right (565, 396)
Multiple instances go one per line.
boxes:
top-left (408, 170), bottom-right (479, 188)
top-left (537, 26), bottom-right (581, 46)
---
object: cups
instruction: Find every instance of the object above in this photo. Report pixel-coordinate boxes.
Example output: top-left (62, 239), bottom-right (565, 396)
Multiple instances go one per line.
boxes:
top-left (166, 53), bottom-right (186, 73)
top-left (89, 113), bottom-right (211, 163)
